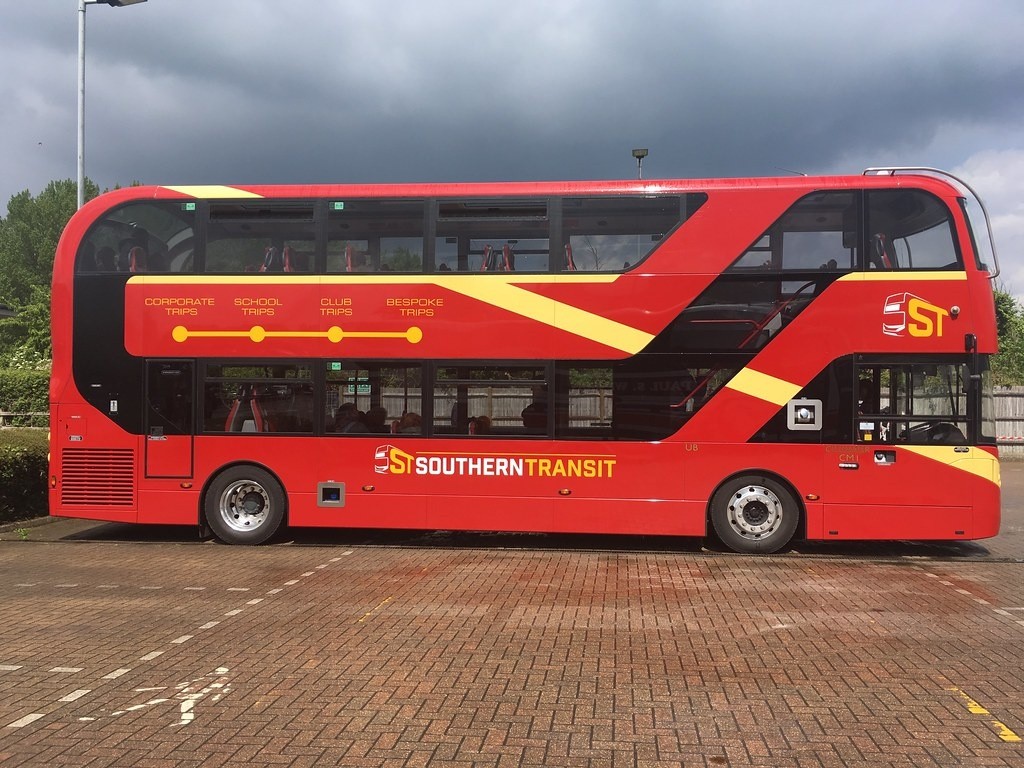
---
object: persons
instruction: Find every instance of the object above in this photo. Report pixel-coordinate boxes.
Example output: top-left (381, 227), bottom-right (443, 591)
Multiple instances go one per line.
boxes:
top-left (396, 412), bottom-right (421, 433)
top-left (521, 375), bottom-right (548, 428)
top-left (335, 402), bottom-right (369, 433)
top-left (77, 228), bottom-right (149, 271)
top-left (856, 379), bottom-right (872, 442)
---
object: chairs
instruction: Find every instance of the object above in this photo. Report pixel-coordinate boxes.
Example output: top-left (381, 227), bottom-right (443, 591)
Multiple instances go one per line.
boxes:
top-left (255, 242), bottom-right (371, 273)
top-left (390, 411), bottom-right (493, 435)
top-left (225, 381), bottom-right (313, 432)
top-left (812, 229), bottom-right (894, 298)
top-left (126, 246), bottom-right (150, 272)
top-left (478, 241), bottom-right (577, 270)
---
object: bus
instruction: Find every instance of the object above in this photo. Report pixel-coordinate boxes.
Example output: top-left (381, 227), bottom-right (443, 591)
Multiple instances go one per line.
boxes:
top-left (45, 165), bottom-right (1000, 559)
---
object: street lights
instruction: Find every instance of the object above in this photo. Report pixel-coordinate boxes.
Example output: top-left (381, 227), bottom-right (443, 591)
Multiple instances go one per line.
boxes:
top-left (631, 148), bottom-right (648, 261)
top-left (76, 0), bottom-right (152, 212)
